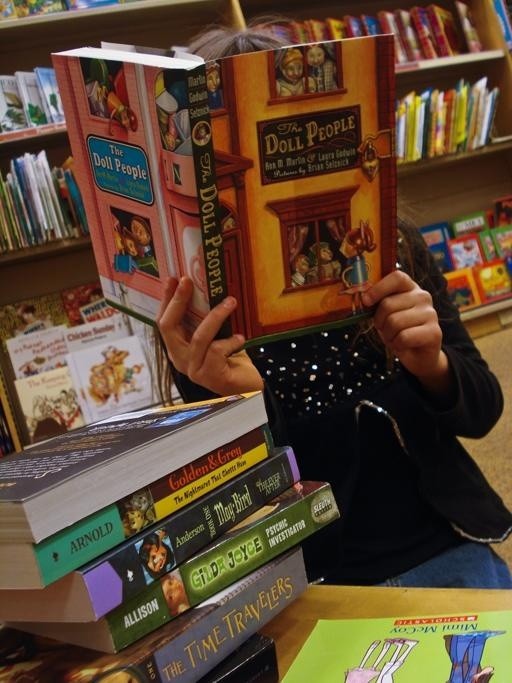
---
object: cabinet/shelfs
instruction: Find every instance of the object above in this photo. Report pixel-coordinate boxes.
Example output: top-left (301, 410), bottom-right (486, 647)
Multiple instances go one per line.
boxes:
top-left (1, 2), bottom-right (512, 339)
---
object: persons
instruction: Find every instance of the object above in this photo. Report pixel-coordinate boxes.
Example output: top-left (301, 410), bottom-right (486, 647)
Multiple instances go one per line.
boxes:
top-left (153, 31), bottom-right (512, 589)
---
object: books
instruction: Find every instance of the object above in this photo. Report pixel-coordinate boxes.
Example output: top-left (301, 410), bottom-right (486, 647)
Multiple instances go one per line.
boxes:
top-left (285, 0), bottom-right (512, 313)
top-left (0, 0), bottom-right (180, 453)
top-left (2, 390), bottom-right (338, 682)
top-left (51, 40), bottom-right (398, 343)
top-left (283, 611), bottom-right (512, 682)
